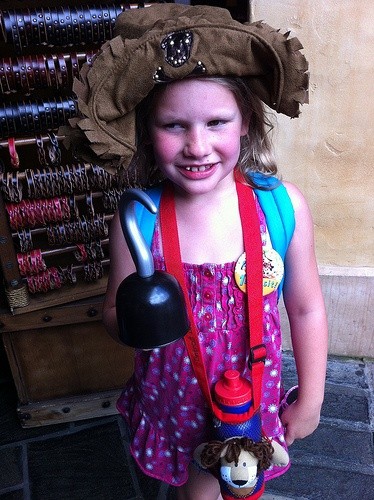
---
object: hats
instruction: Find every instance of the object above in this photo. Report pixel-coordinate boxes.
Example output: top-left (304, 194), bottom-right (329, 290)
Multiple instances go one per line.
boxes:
top-left (57, 3), bottom-right (310, 175)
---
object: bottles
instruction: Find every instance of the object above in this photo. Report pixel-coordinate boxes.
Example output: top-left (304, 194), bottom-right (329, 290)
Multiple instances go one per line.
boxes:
top-left (209, 371), bottom-right (265, 500)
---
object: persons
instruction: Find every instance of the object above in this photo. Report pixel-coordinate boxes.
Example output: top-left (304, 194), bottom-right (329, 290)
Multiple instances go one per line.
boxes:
top-left (59, 3), bottom-right (328, 500)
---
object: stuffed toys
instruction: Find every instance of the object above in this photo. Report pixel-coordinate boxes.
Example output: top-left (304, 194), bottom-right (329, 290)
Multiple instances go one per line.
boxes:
top-left (194, 436), bottom-right (289, 500)
